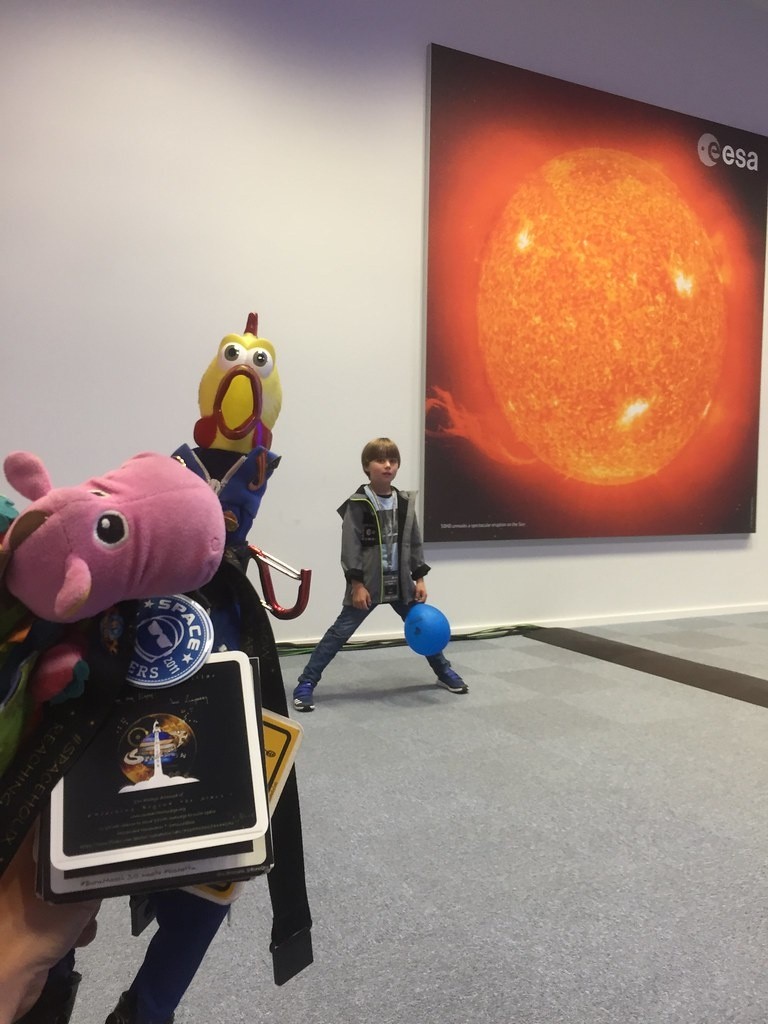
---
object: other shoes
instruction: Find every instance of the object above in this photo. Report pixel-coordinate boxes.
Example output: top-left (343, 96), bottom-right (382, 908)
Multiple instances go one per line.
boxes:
top-left (106, 992), bottom-right (176, 1024)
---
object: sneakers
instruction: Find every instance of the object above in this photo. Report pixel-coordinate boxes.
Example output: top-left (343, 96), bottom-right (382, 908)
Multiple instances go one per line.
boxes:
top-left (293, 681), bottom-right (315, 712)
top-left (437, 669), bottom-right (469, 693)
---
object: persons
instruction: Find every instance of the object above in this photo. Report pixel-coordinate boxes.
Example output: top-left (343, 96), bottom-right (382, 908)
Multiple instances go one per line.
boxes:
top-left (293, 437), bottom-right (470, 712)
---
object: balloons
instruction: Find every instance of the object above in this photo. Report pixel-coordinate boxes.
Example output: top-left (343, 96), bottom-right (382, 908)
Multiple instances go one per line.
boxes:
top-left (404, 602), bottom-right (451, 656)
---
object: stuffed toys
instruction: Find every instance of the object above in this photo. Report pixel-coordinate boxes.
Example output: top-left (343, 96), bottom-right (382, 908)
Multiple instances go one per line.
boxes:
top-left (0, 450), bottom-right (226, 624)
